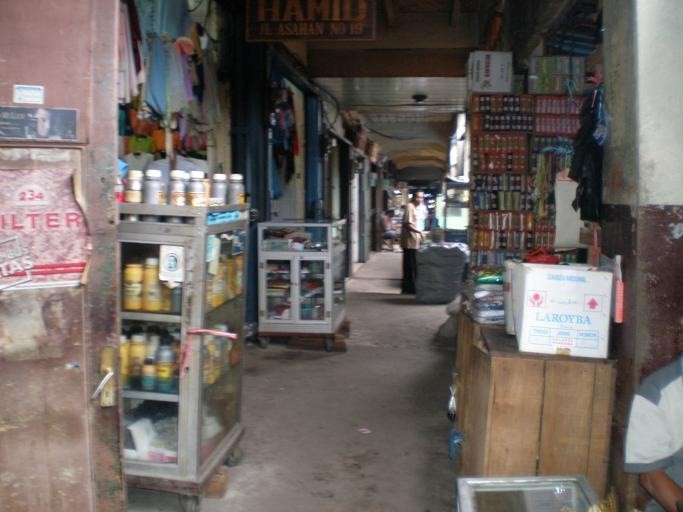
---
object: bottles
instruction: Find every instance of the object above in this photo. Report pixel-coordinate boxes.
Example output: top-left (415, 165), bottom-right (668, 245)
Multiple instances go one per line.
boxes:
top-left (212, 172), bottom-right (245, 205)
top-left (142, 168), bottom-right (166, 222)
top-left (124, 169), bottom-right (144, 223)
top-left (168, 169), bottom-right (211, 225)
top-left (202, 324), bottom-right (232, 386)
top-left (205, 252), bottom-right (243, 308)
top-left (123, 257), bottom-right (181, 313)
top-left (120, 326), bottom-right (179, 393)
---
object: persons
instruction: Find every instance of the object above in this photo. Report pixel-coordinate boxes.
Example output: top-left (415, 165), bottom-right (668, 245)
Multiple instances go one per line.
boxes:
top-left (620, 354), bottom-right (682, 512)
top-left (381, 204), bottom-right (407, 240)
top-left (25, 109), bottom-right (63, 141)
top-left (423, 210), bottom-right (438, 231)
top-left (399, 188), bottom-right (427, 296)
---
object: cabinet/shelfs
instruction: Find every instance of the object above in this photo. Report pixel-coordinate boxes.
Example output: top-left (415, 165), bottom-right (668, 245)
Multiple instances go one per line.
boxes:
top-left (453, 310), bottom-right (616, 512)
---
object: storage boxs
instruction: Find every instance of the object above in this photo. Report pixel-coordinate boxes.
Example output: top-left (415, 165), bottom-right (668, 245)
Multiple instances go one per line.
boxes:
top-left (505, 259), bottom-right (614, 360)
